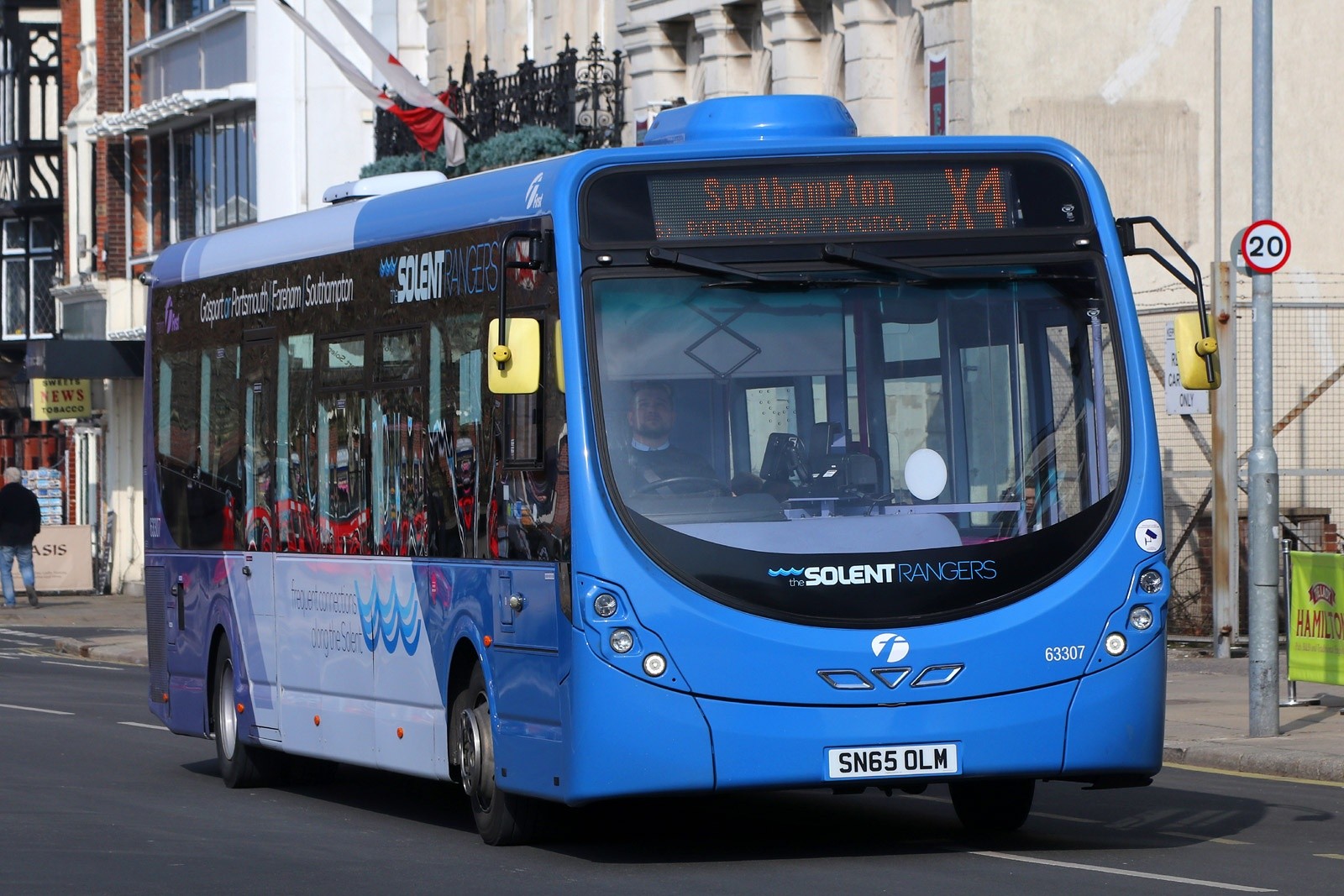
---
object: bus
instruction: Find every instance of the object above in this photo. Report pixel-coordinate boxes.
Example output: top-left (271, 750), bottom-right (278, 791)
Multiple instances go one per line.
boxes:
top-left (140, 96), bottom-right (1222, 847)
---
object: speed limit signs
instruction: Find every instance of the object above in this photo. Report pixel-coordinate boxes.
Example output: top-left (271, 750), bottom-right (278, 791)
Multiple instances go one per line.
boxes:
top-left (1241, 219), bottom-right (1292, 273)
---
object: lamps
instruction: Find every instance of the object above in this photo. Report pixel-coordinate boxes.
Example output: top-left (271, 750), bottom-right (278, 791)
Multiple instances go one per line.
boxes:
top-left (78, 234), bottom-right (99, 257)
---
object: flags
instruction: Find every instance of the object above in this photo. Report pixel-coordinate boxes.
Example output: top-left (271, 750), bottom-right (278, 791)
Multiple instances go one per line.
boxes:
top-left (272, 1), bottom-right (471, 169)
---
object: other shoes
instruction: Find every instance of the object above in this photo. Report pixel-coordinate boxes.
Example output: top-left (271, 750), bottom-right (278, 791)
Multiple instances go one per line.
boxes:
top-left (0, 602), bottom-right (13, 608)
top-left (27, 589), bottom-right (39, 606)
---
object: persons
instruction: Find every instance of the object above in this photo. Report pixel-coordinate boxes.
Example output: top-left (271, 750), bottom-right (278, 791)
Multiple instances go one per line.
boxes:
top-left (0, 466), bottom-right (41, 607)
top-left (993, 476), bottom-right (1038, 528)
top-left (609, 383), bottom-right (736, 501)
